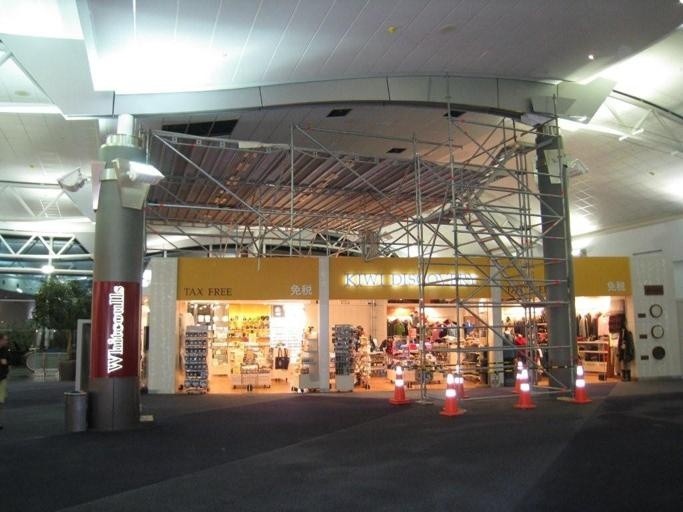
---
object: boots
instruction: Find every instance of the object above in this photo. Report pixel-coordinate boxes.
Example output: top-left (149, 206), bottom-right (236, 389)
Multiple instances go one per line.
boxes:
top-left (620, 369), bottom-right (632, 382)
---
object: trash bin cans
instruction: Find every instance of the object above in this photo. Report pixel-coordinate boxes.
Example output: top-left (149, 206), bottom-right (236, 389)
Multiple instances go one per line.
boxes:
top-left (64, 390), bottom-right (89, 437)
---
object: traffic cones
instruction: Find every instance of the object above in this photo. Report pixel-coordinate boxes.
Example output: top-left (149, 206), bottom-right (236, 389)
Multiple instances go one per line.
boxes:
top-left (512, 361), bottom-right (536, 410)
top-left (570, 365), bottom-right (592, 404)
top-left (389, 365), bottom-right (412, 404)
top-left (440, 372), bottom-right (468, 415)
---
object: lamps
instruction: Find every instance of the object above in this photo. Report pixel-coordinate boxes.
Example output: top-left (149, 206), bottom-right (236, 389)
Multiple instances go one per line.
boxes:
top-left (100, 159), bottom-right (119, 181)
top-left (126, 162), bottom-right (165, 184)
top-left (565, 159), bottom-right (588, 177)
top-left (58, 168), bottom-right (91, 192)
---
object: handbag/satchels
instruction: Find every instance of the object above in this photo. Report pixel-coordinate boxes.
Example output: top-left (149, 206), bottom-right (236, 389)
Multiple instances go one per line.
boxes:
top-left (275, 347), bottom-right (290, 370)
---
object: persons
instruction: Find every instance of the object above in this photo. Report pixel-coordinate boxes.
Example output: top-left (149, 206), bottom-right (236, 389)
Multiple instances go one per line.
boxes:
top-left (0, 333), bottom-right (15, 429)
top-left (527, 331), bottom-right (544, 374)
top-left (617, 326), bottom-right (632, 382)
top-left (515, 333), bottom-right (527, 361)
top-left (387, 319), bottom-right (475, 338)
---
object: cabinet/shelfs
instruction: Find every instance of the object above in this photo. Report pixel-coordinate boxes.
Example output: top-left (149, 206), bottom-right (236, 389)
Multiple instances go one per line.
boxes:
top-left (184, 302), bottom-right (470, 390)
